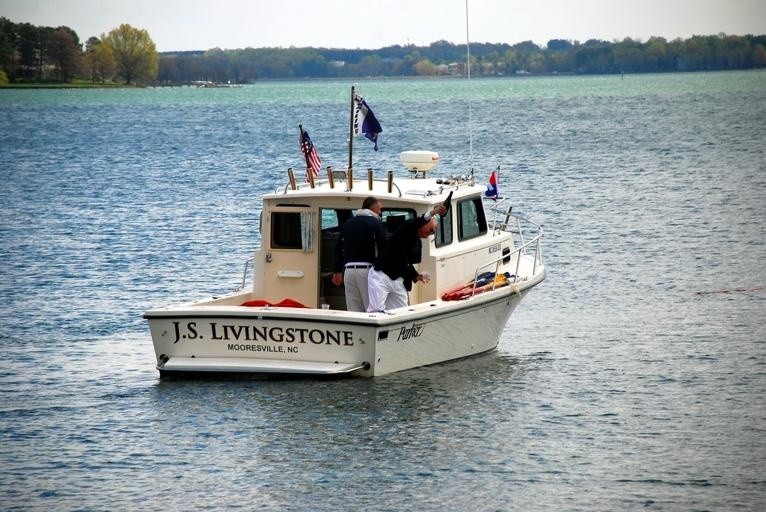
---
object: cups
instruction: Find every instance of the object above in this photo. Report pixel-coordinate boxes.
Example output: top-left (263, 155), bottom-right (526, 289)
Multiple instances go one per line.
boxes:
top-left (422, 272), bottom-right (429, 282)
top-left (321, 304), bottom-right (330, 314)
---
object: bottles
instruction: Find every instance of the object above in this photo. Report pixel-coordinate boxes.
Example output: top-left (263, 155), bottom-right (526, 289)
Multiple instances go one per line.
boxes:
top-left (438, 190), bottom-right (453, 217)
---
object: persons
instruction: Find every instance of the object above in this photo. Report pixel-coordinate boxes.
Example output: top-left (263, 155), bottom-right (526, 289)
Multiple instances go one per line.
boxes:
top-left (332, 196), bottom-right (383, 311)
top-left (366, 204), bottom-right (448, 313)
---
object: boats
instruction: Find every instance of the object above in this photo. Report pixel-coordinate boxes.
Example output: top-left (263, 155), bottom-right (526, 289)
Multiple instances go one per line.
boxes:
top-left (140, 151), bottom-right (549, 381)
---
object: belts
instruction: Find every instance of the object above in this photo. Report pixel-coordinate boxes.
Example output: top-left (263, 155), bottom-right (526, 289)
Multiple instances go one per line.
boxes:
top-left (345, 265), bottom-right (370, 268)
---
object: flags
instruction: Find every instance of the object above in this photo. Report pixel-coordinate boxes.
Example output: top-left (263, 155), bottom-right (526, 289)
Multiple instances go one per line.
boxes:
top-left (299, 130), bottom-right (322, 183)
top-left (485, 172), bottom-right (498, 202)
top-left (352, 91), bottom-right (383, 151)
